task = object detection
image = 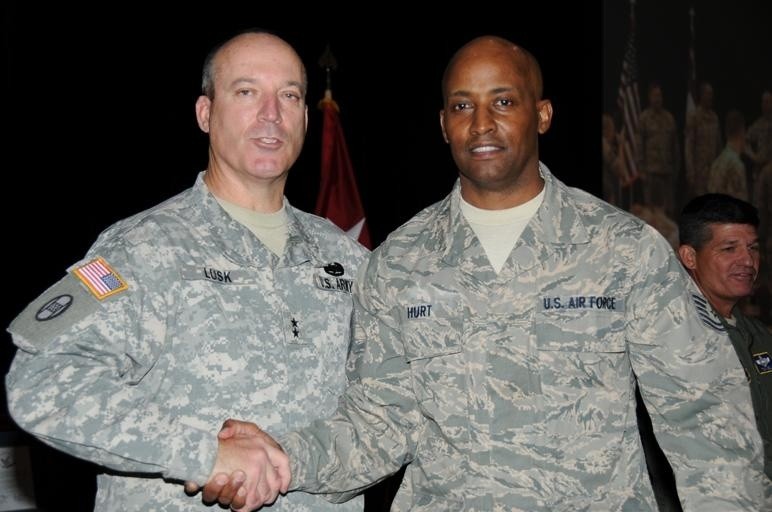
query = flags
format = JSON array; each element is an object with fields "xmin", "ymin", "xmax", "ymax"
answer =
[
  {"xmin": 309, "ymin": 87, "xmax": 373, "ymax": 250},
  {"xmin": 617, "ymin": 43, "xmax": 645, "ymax": 188}
]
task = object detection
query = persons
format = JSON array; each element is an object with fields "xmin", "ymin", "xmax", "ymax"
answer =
[
  {"xmin": 185, "ymin": 35, "xmax": 772, "ymax": 512},
  {"xmin": 638, "ymin": 191, "xmax": 772, "ymax": 511},
  {"xmin": 599, "ymin": 80, "xmax": 772, "ymax": 271},
  {"xmin": 5, "ymin": 31, "xmax": 372, "ymax": 512}
]
